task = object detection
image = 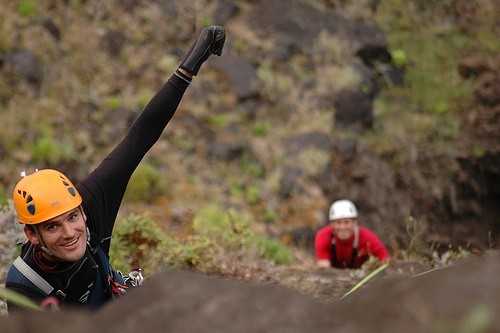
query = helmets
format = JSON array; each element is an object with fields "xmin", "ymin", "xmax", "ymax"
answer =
[
  {"xmin": 329, "ymin": 199, "xmax": 359, "ymax": 222},
  {"xmin": 13, "ymin": 168, "xmax": 83, "ymax": 224}
]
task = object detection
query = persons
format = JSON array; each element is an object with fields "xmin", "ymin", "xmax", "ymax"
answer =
[
  {"xmin": 4, "ymin": 23, "xmax": 226, "ymax": 317},
  {"xmin": 314, "ymin": 199, "xmax": 392, "ymax": 270}
]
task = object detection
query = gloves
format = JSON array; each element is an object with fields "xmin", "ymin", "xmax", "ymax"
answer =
[{"xmin": 179, "ymin": 25, "xmax": 227, "ymax": 77}]
